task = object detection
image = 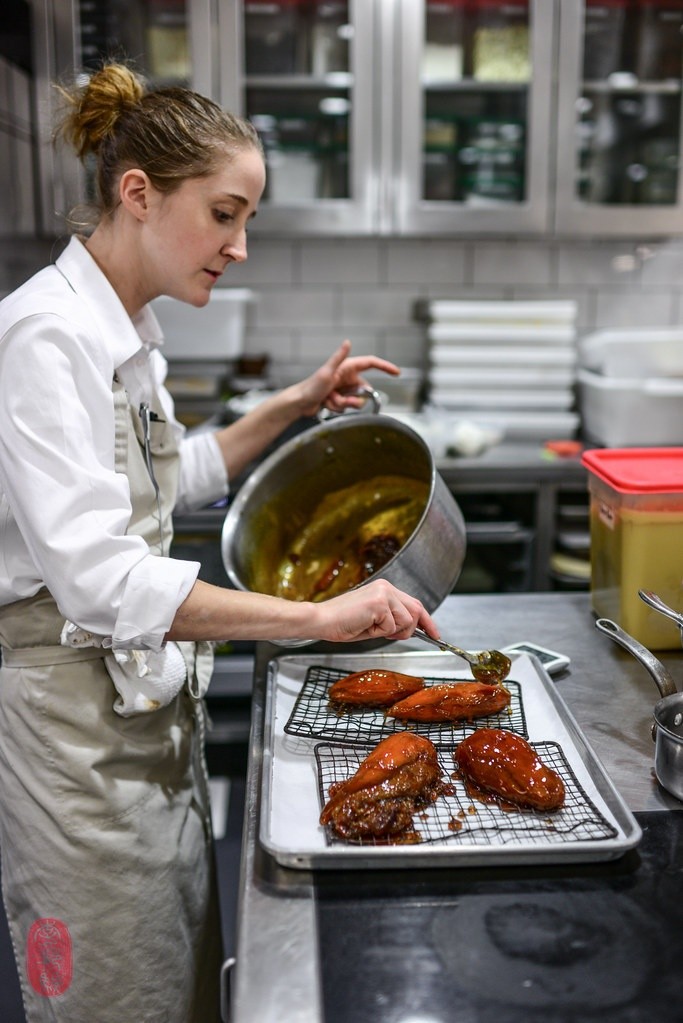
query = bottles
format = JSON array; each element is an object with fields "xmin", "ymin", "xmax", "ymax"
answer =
[
  {"xmin": 251, "ymin": 116, "xmax": 349, "ymax": 199},
  {"xmin": 470, "ymin": 125, "xmax": 521, "ymax": 196}
]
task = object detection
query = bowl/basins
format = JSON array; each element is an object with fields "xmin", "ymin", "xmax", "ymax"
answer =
[{"xmin": 227, "ymin": 384, "xmax": 387, "ymax": 463}]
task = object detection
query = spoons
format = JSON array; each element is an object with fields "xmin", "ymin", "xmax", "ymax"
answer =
[{"xmin": 413, "ymin": 628, "xmax": 511, "ymax": 685}]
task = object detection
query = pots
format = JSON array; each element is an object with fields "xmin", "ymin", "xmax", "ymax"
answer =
[
  {"xmin": 596, "ymin": 618, "xmax": 683, "ymax": 799},
  {"xmin": 220, "ymin": 386, "xmax": 467, "ymax": 649}
]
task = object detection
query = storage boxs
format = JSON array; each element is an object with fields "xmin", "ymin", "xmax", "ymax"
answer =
[
  {"xmin": 150, "ymin": 289, "xmax": 258, "ymax": 357},
  {"xmin": 581, "ymin": 328, "xmax": 683, "ymax": 648}
]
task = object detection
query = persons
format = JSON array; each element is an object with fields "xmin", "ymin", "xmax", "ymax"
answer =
[{"xmin": 1, "ymin": 64, "xmax": 448, "ymax": 1023}]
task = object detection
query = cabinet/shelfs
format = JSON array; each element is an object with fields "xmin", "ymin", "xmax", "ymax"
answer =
[
  {"xmin": 34, "ymin": 0, "xmax": 384, "ymax": 250},
  {"xmin": 376, "ymin": 0, "xmax": 683, "ymax": 241},
  {"xmin": 427, "ymin": 436, "xmax": 606, "ymax": 596}
]
